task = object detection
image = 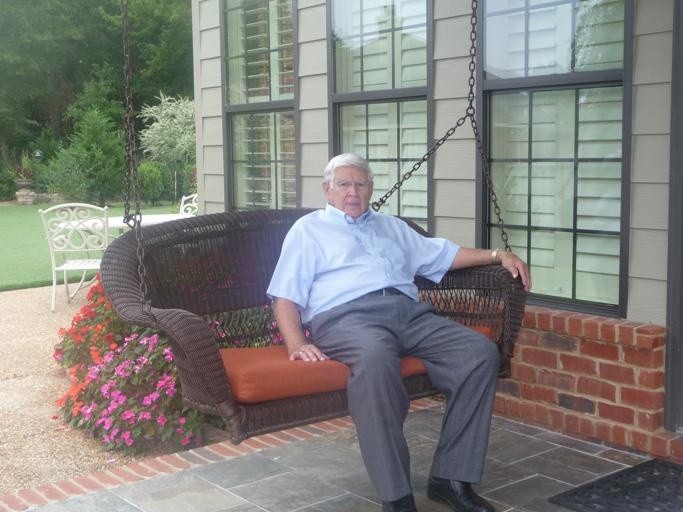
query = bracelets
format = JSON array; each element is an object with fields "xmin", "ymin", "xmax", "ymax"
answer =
[{"xmin": 492, "ymin": 246, "xmax": 503, "ymax": 265}]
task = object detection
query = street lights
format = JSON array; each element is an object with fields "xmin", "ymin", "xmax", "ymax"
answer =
[{"xmin": 31, "ymin": 150, "xmax": 44, "ymax": 202}]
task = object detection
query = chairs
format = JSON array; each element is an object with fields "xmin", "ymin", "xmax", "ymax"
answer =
[
  {"xmin": 179, "ymin": 194, "xmax": 198, "ymax": 214},
  {"xmin": 37, "ymin": 203, "xmax": 123, "ymax": 315}
]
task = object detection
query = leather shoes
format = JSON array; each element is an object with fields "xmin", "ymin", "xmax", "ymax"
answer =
[
  {"xmin": 428, "ymin": 473, "xmax": 496, "ymax": 512},
  {"xmin": 383, "ymin": 493, "xmax": 417, "ymax": 512}
]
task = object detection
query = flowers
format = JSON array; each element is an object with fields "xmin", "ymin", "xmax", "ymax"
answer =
[{"xmin": 15, "ymin": 165, "xmax": 33, "ymax": 180}]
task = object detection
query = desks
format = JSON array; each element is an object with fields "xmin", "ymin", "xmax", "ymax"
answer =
[{"xmin": 60, "ymin": 213, "xmax": 197, "ymax": 301}]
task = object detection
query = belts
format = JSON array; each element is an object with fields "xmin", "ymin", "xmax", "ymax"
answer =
[{"xmin": 366, "ymin": 287, "xmax": 403, "ymax": 297}]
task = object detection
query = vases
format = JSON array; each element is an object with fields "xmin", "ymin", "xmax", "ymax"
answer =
[{"xmin": 16, "ymin": 180, "xmax": 32, "ymax": 188}]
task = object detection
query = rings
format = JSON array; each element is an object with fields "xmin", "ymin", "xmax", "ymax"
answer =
[{"xmin": 298, "ymin": 350, "xmax": 304, "ymax": 356}]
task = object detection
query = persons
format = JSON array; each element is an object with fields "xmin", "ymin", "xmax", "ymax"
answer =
[{"xmin": 266, "ymin": 153, "xmax": 532, "ymax": 511}]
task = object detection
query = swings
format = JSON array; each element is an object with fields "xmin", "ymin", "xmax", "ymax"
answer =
[{"xmin": 98, "ymin": 0, "xmax": 529, "ymax": 445}]
